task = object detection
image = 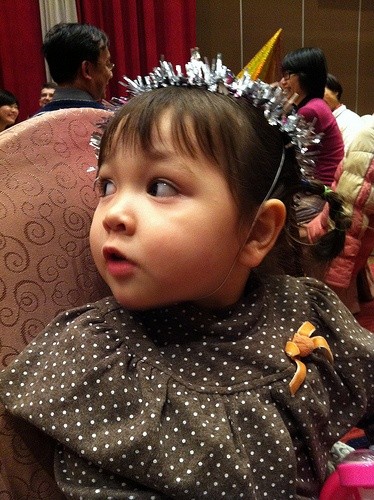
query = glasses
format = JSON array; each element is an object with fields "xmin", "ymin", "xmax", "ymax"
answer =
[
  {"xmin": 280, "ymin": 71, "xmax": 294, "ymax": 80},
  {"xmin": 91, "ymin": 60, "xmax": 115, "ymax": 71}
]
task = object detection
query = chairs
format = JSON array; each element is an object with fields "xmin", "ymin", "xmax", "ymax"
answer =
[
  {"xmin": 0, "ymin": 109, "xmax": 112, "ymax": 500},
  {"xmin": 311, "ymin": 114, "xmax": 374, "ymax": 317}
]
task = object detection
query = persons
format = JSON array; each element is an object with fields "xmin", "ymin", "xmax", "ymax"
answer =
[
  {"xmin": 1, "ymin": 22, "xmax": 374, "ymax": 226},
  {"xmin": 1, "ymin": 47, "xmax": 374, "ymax": 500}
]
